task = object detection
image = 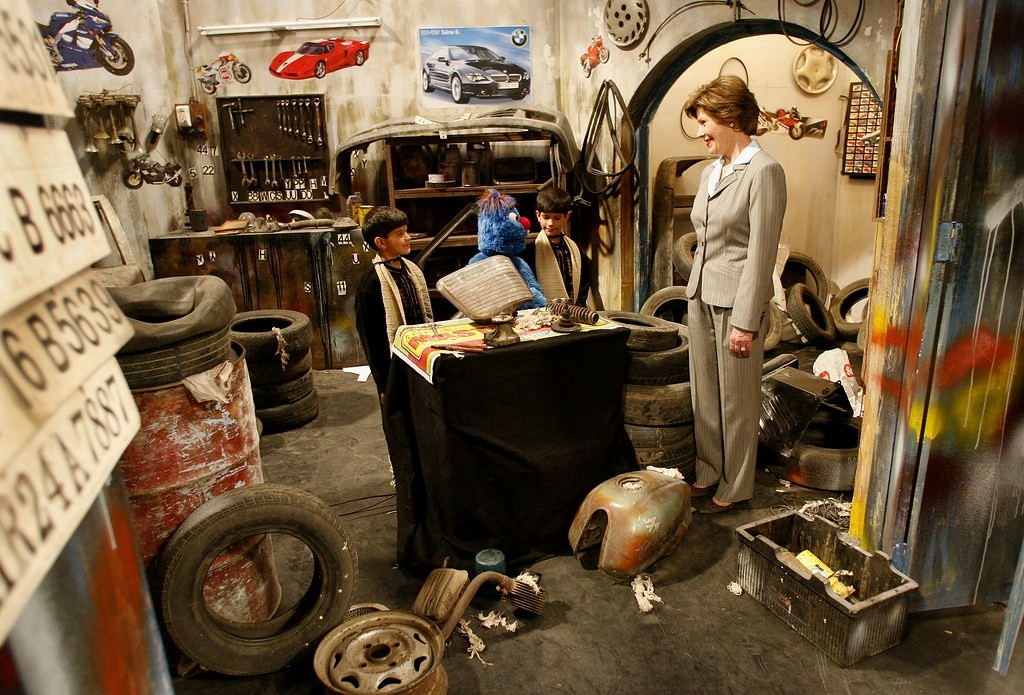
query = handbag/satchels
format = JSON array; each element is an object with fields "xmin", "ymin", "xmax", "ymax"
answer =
[{"xmin": 812, "ymin": 348, "xmax": 864, "ymax": 417}]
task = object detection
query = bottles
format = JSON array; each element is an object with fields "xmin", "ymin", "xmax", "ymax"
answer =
[
  {"xmin": 467, "ymin": 141, "xmax": 494, "ymax": 185},
  {"xmin": 437, "ymin": 144, "xmax": 460, "ymax": 182},
  {"xmin": 347, "ymin": 192, "xmax": 363, "ymax": 223},
  {"xmin": 462, "ymin": 162, "xmax": 478, "ymax": 186}
]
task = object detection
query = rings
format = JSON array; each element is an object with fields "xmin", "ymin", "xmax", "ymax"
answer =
[{"xmin": 741, "ymin": 347, "xmax": 746, "ymax": 351}]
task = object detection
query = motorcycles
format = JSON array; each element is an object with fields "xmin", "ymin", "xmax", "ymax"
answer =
[
  {"xmin": 35, "ymin": 0, "xmax": 135, "ymax": 75},
  {"xmin": 758, "ymin": 107, "xmax": 806, "ymax": 139},
  {"xmin": 123, "ymin": 155, "xmax": 182, "ymax": 189},
  {"xmin": 193, "ymin": 53, "xmax": 251, "ymax": 95},
  {"xmin": 581, "ymin": 37, "xmax": 610, "ymax": 77}
]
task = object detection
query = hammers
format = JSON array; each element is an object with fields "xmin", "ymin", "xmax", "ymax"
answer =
[{"xmin": 223, "ymin": 103, "xmax": 236, "ymax": 130}]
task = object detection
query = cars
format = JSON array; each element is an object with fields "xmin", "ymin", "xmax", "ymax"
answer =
[{"xmin": 422, "ymin": 44, "xmax": 530, "ymax": 104}]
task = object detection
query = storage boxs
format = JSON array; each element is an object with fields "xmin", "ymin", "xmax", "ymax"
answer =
[{"xmin": 735, "ymin": 510, "xmax": 921, "ymax": 667}]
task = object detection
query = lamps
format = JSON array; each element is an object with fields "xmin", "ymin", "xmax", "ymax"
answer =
[{"xmin": 196, "ymin": 16, "xmax": 381, "ymax": 36}]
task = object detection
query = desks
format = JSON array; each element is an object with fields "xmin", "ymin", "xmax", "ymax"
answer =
[{"xmin": 391, "ymin": 316, "xmax": 631, "ymax": 581}]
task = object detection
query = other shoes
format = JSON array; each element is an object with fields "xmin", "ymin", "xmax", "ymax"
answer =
[
  {"xmin": 689, "ymin": 483, "xmax": 717, "ymax": 496},
  {"xmin": 699, "ymin": 496, "xmax": 734, "ymax": 513}
]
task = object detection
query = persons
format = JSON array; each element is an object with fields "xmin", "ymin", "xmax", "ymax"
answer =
[
  {"xmin": 353, "ymin": 205, "xmax": 436, "ymax": 412},
  {"xmin": 683, "ymin": 74, "xmax": 787, "ymax": 515},
  {"xmin": 519, "ymin": 187, "xmax": 593, "ymax": 310}
]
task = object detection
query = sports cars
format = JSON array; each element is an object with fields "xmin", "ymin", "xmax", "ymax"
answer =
[{"xmin": 269, "ymin": 36, "xmax": 370, "ymax": 79}]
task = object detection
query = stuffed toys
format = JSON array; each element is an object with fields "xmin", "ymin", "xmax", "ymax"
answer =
[{"xmin": 468, "ymin": 188, "xmax": 548, "ymax": 311}]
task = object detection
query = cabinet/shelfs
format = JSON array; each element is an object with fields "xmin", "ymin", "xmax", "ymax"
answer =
[
  {"xmin": 145, "ymin": 216, "xmax": 382, "ymax": 372},
  {"xmin": 383, "ymin": 132, "xmax": 571, "ymax": 322}
]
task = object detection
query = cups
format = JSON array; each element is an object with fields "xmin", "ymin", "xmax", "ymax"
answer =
[{"xmin": 359, "ymin": 206, "xmax": 374, "ymax": 227}]
